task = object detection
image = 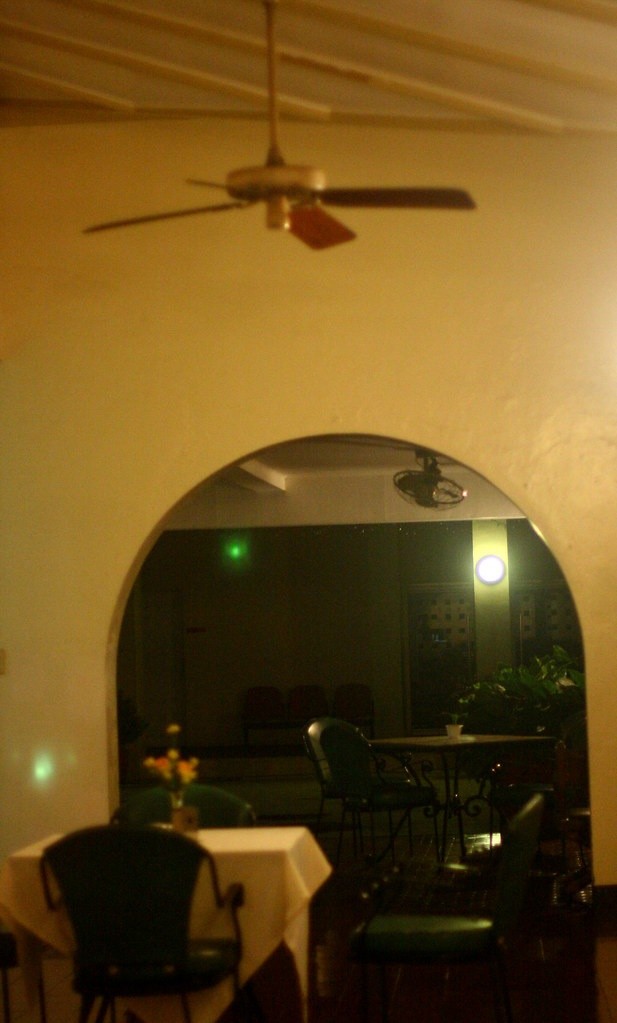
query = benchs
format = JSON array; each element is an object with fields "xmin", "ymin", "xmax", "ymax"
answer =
[{"xmin": 239, "ymin": 685, "xmax": 375, "ymax": 758}]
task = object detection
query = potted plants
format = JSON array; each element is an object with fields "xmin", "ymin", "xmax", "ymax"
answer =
[
  {"xmin": 451, "ymin": 640, "xmax": 586, "ymax": 841},
  {"xmin": 440, "ymin": 711, "xmax": 469, "ymax": 739}
]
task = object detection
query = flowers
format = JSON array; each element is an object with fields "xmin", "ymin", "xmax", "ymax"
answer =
[{"xmin": 141, "ymin": 722, "xmax": 200, "ymax": 793}]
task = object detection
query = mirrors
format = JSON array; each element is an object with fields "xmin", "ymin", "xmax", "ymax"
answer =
[{"xmin": 116, "ymin": 433, "xmax": 594, "ymax": 1023}]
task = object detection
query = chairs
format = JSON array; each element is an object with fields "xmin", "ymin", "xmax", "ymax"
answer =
[
  {"xmin": 344, "ymin": 812, "xmax": 541, "ymax": 1023},
  {"xmin": 299, "ymin": 717, "xmax": 439, "ymax": 871},
  {"xmin": 39, "ymin": 828, "xmax": 247, "ymax": 1023},
  {"xmin": 0, "ymin": 931, "xmax": 47, "ymax": 1023},
  {"xmin": 110, "ymin": 781, "xmax": 258, "ymax": 827},
  {"xmin": 484, "ymin": 722, "xmax": 582, "ymax": 862}
]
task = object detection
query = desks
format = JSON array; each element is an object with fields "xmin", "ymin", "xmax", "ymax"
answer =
[
  {"xmin": 367, "ymin": 733, "xmax": 557, "ymax": 866},
  {"xmin": 0, "ymin": 824, "xmax": 334, "ymax": 1023}
]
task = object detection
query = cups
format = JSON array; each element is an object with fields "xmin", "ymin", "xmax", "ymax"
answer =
[{"xmin": 446, "ymin": 725, "xmax": 463, "ymax": 741}]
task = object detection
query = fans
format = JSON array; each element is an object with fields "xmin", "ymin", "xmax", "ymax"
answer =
[
  {"xmin": 393, "ymin": 447, "xmax": 466, "ymax": 511},
  {"xmin": 80, "ymin": 0, "xmax": 478, "ymax": 250}
]
